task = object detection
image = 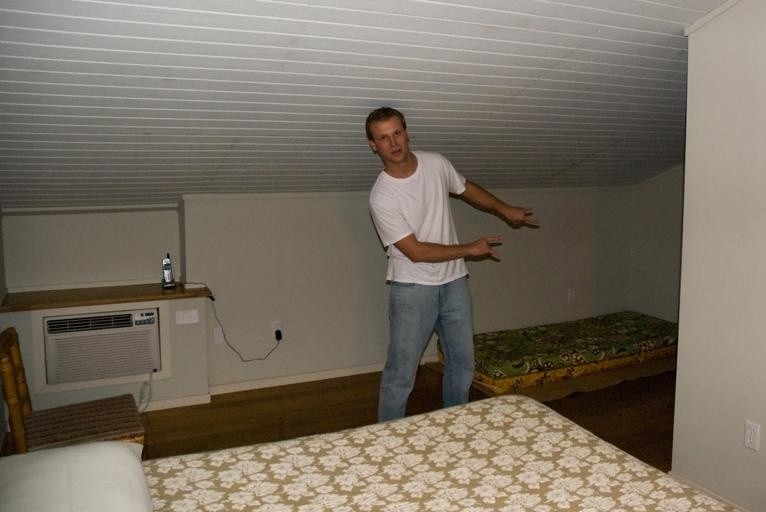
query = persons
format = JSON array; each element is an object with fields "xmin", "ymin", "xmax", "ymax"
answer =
[{"xmin": 366, "ymin": 107, "xmax": 542, "ymax": 424}]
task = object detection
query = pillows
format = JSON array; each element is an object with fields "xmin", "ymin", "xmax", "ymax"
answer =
[{"xmin": 0, "ymin": 438, "xmax": 154, "ymax": 512}]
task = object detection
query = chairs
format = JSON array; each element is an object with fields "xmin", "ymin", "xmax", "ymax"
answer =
[{"xmin": 0, "ymin": 326, "xmax": 146, "ymax": 457}]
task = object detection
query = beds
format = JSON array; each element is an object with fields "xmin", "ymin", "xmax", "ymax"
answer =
[
  {"xmin": 141, "ymin": 394, "xmax": 745, "ymax": 512},
  {"xmin": 435, "ymin": 312, "xmax": 677, "ymax": 404}
]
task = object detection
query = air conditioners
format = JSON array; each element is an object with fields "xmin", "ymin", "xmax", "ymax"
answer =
[{"xmin": 43, "ymin": 308, "xmax": 161, "ymax": 385}]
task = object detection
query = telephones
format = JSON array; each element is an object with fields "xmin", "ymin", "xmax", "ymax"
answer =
[{"xmin": 161, "ymin": 253, "xmax": 176, "ymax": 289}]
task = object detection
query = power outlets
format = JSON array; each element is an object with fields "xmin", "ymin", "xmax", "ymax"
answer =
[
  {"xmin": 745, "ymin": 420, "xmax": 761, "ymax": 450},
  {"xmin": 272, "ymin": 320, "xmax": 284, "ymax": 345}
]
